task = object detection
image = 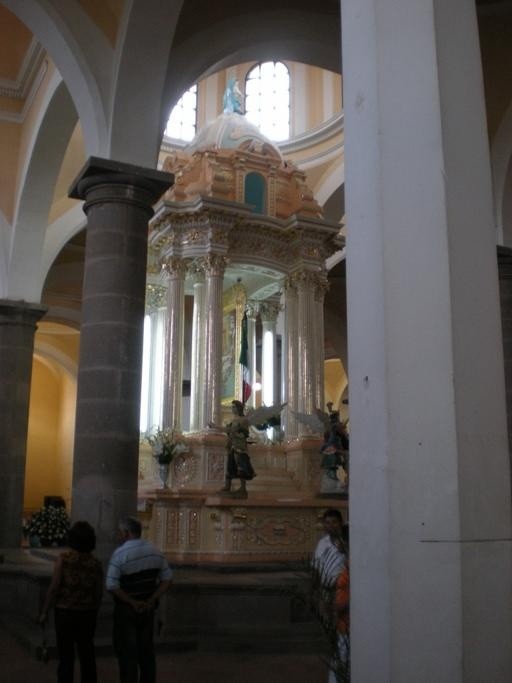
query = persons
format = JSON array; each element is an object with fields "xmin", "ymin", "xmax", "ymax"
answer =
[
  {"xmin": 309, "ymin": 505, "xmax": 341, "ymax": 583},
  {"xmin": 35, "ymin": 521, "xmax": 106, "ymax": 683},
  {"xmin": 322, "ymin": 411, "xmax": 346, "ymax": 481},
  {"xmin": 313, "ymin": 522, "xmax": 349, "ymax": 647},
  {"xmin": 208, "ymin": 399, "xmax": 255, "ymax": 498},
  {"xmin": 104, "ymin": 514, "xmax": 175, "ymax": 683}
]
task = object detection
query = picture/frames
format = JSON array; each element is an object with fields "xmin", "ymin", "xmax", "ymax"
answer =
[{"xmin": 220, "ymin": 301, "xmax": 243, "ymax": 406}]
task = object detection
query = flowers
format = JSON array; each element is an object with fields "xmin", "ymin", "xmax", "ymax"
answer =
[{"xmin": 143, "ymin": 425, "xmax": 190, "ymax": 463}]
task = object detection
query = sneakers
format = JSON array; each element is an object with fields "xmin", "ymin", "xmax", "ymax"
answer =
[{"xmin": 216, "ymin": 486, "xmax": 249, "ymax": 499}]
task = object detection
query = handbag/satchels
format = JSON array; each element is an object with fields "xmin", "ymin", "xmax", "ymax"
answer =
[
  {"xmin": 225, "ymin": 453, "xmax": 253, "ymax": 480},
  {"xmin": 37, "ymin": 562, "xmax": 72, "ymax": 609}
]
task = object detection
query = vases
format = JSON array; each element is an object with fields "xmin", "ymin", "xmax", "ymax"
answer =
[{"xmin": 153, "ymin": 454, "xmax": 173, "ymax": 489}]
